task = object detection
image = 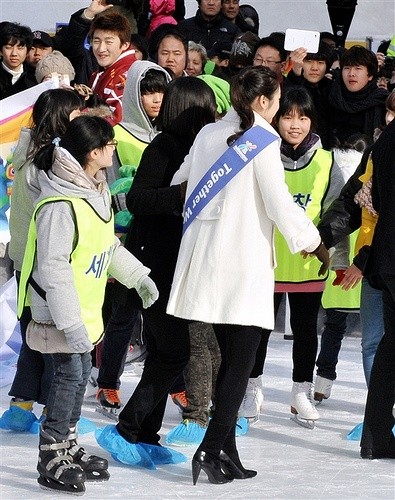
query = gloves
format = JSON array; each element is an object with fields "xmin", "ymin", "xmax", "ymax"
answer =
[
  {"xmin": 136, "ymin": 278, "xmax": 159, "ymax": 309},
  {"xmin": 332, "ymin": 270, "xmax": 347, "ymax": 286},
  {"xmin": 65, "ymin": 324, "xmax": 94, "ymax": 353}
]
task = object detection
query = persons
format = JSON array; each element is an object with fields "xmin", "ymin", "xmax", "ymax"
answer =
[
  {"xmin": 166, "ymin": 66, "xmax": 329, "ymax": 485},
  {"xmin": 95, "ymin": 76, "xmax": 220, "ymax": 471},
  {"xmin": 360, "ymin": 119, "xmax": 395, "ymax": 460},
  {"xmin": 17, "ymin": 116, "xmax": 159, "ymax": 495},
  {"xmin": 238, "ymin": 87, "xmax": 351, "ymax": 430},
  {"xmin": 313, "ymin": 137, "xmax": 374, "ymax": 406},
  {"xmin": 300, "ymin": 86, "xmax": 395, "ymax": 441},
  {"xmin": 255, "ymin": 32, "xmax": 395, "ymax": 340},
  {"xmin": 0, "ymin": 0, "xmax": 248, "ymax": 443}
]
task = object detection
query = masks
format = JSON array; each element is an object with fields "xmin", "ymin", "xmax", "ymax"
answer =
[
  {"xmin": 204, "ymin": 60, "xmax": 222, "ymax": 76},
  {"xmin": 228, "ymin": 66, "xmax": 245, "ymax": 76}
]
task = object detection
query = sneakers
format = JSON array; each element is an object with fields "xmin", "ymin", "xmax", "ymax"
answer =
[
  {"xmin": 289, "ymin": 381, "xmax": 319, "ymax": 430},
  {"xmin": 36, "ymin": 420, "xmax": 111, "ymax": 496},
  {"xmin": 171, "ymin": 391, "xmax": 188, "ymax": 419},
  {"xmin": 192, "ymin": 448, "xmax": 257, "ymax": 484},
  {"xmin": 95, "ymin": 387, "xmax": 125, "ymax": 422},
  {"xmin": 237, "ymin": 374, "xmax": 264, "ymax": 425},
  {"xmin": 314, "ymin": 375, "xmax": 334, "ymax": 405}
]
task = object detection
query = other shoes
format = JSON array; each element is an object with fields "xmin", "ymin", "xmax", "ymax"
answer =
[
  {"xmin": 10, "ymin": 398, "xmax": 35, "ymax": 431},
  {"xmin": 360, "ymin": 445, "xmax": 395, "ymax": 459}
]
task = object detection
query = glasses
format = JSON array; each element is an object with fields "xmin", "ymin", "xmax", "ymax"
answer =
[
  {"xmin": 253, "ymin": 57, "xmax": 283, "ymax": 66},
  {"xmin": 105, "ymin": 139, "xmax": 118, "ymax": 147}
]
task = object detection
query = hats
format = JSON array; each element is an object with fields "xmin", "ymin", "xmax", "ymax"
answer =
[
  {"xmin": 230, "ymin": 31, "xmax": 260, "ymax": 66},
  {"xmin": 31, "ymin": 30, "xmax": 53, "ymax": 47},
  {"xmin": 207, "ymin": 40, "xmax": 232, "ymax": 58},
  {"xmin": 35, "ymin": 50, "xmax": 75, "ymax": 83}
]
